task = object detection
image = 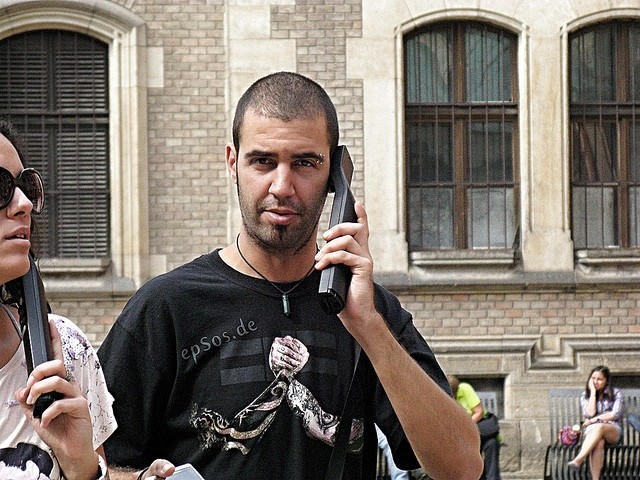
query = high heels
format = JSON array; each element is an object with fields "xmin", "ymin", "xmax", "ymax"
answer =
[{"xmin": 568, "ymin": 461, "xmax": 579, "ymax": 475}]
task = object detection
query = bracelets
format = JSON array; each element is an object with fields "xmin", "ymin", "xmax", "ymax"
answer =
[{"xmin": 136, "ymin": 466, "xmax": 149, "ymax": 480}]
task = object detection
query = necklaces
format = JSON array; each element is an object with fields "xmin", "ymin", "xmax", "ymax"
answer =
[{"xmin": 236, "ymin": 232, "xmax": 318, "ymax": 316}]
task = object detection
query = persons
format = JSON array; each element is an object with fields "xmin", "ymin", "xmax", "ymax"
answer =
[
  {"xmin": 568, "ymin": 366, "xmax": 622, "ymax": 480},
  {"xmin": 444, "ymin": 375, "xmax": 503, "ymax": 480},
  {"xmin": 1, "ymin": 117, "xmax": 108, "ymax": 480},
  {"xmin": 96, "ymin": 72, "xmax": 484, "ymax": 480}
]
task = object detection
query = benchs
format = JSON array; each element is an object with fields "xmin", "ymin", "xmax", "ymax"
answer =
[{"xmin": 543, "ymin": 389, "xmax": 639, "ymax": 476}]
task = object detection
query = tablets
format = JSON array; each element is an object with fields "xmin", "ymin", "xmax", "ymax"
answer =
[{"xmin": 138, "ymin": 462, "xmax": 205, "ymax": 480}]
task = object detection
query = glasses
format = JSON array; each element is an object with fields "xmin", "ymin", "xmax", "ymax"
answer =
[{"xmin": 0, "ymin": 167, "xmax": 44, "ymax": 215}]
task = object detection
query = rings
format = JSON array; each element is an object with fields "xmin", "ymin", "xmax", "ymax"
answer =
[{"xmin": 64, "ymin": 364, "xmax": 76, "ymax": 383}]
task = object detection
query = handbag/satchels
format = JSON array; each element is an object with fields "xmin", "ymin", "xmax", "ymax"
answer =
[
  {"xmin": 559, "ymin": 424, "xmax": 581, "ymax": 446},
  {"xmin": 477, "ymin": 412, "xmax": 500, "ymax": 439}
]
